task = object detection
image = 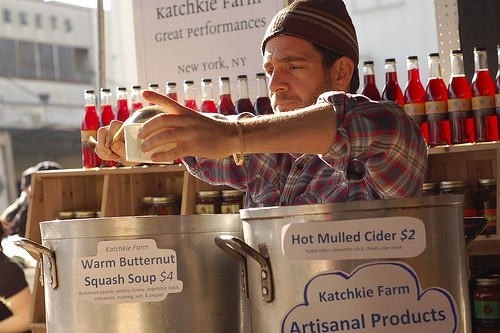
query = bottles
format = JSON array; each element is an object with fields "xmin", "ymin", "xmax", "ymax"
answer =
[
  {"xmin": 234, "ymin": 74, "xmax": 254, "ymax": 115},
  {"xmin": 141, "ymin": 194, "xmax": 180, "ymax": 215},
  {"xmin": 360, "ymin": 60, "xmax": 381, "ymax": 102},
  {"xmin": 166, "ymin": 82, "xmax": 177, "ymax": 101},
  {"xmin": 470, "ymin": 45, "xmax": 498, "ymax": 142},
  {"xmin": 253, "ymin": 72, "xmax": 274, "ymax": 115},
  {"xmin": 183, "ymin": 80, "xmax": 199, "ymax": 112},
  {"xmin": 494, "ymin": 45, "xmax": 500, "ymax": 140},
  {"xmin": 381, "ymin": 58, "xmax": 404, "ymax": 105},
  {"xmin": 199, "ymin": 78, "xmax": 218, "ymax": 113},
  {"xmin": 425, "ymin": 53, "xmax": 451, "ymax": 147},
  {"xmin": 420, "ymin": 177, "xmax": 500, "ymax": 333},
  {"xmin": 81, "ymin": 84, "xmax": 159, "ymax": 168},
  {"xmin": 447, "ymin": 49, "xmax": 475, "ymax": 145},
  {"xmin": 403, "ymin": 55, "xmax": 430, "ymax": 147},
  {"xmin": 216, "ymin": 77, "xmax": 234, "ymax": 115},
  {"xmin": 194, "ymin": 189, "xmax": 244, "ymax": 213}
]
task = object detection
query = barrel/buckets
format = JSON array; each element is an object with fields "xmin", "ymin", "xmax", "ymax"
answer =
[
  {"xmin": 214, "ymin": 192, "xmax": 488, "ymax": 333},
  {"xmin": 12, "ymin": 213, "xmax": 240, "ymax": 333}
]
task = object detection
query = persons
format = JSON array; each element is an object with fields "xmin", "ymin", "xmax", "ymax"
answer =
[
  {"xmin": 94, "ymin": 0, "xmax": 427, "ymax": 208},
  {"xmin": 0, "ymin": 161, "xmax": 64, "ymax": 333}
]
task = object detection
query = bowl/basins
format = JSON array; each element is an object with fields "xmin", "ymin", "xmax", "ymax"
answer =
[{"xmin": 123, "ymin": 122, "xmax": 174, "ymax": 166}]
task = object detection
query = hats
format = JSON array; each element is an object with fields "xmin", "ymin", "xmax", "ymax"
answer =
[{"xmin": 261, "ymin": 0, "xmax": 359, "ymax": 69}]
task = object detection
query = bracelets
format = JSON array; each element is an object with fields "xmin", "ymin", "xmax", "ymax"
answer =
[{"xmin": 232, "ymin": 121, "xmax": 244, "ymax": 165}]
